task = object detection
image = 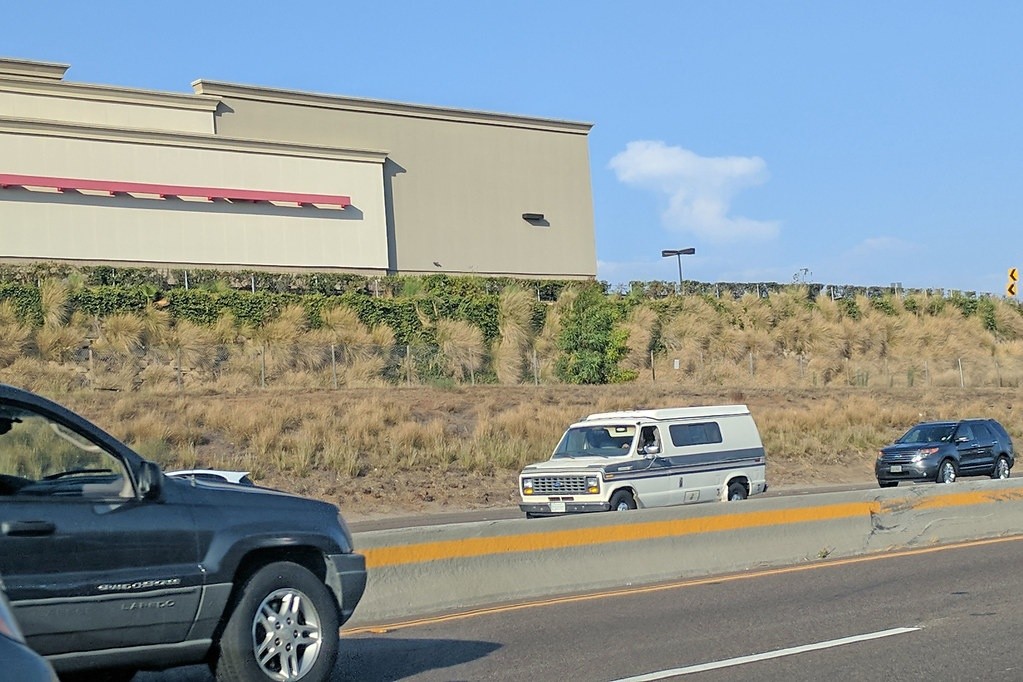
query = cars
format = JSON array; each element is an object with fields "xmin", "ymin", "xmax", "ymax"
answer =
[{"xmin": 0, "ymin": 578, "xmax": 59, "ymax": 679}]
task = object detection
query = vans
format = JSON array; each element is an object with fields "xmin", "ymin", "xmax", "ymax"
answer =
[{"xmin": 517, "ymin": 403, "xmax": 769, "ymax": 520}]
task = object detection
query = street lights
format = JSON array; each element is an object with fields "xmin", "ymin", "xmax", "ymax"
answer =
[{"xmin": 662, "ymin": 247, "xmax": 694, "ymax": 292}]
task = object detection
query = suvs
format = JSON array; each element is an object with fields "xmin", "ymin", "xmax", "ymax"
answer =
[
  {"xmin": 875, "ymin": 418, "xmax": 1016, "ymax": 489},
  {"xmin": 1, "ymin": 384, "xmax": 367, "ymax": 682}
]
